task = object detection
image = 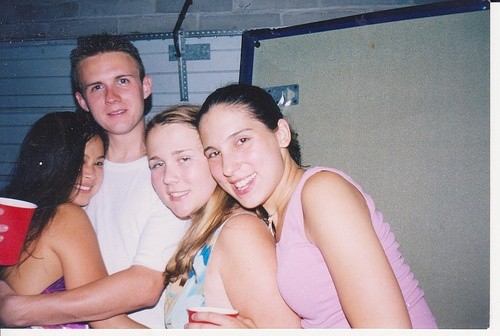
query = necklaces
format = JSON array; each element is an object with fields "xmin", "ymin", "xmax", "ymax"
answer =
[{"xmin": 266, "ymin": 166, "xmax": 297, "ymax": 222}]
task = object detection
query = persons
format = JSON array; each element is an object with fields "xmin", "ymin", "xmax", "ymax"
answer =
[
  {"xmin": 0, "ymin": 33, "xmax": 192, "ymax": 328},
  {"xmin": 142, "ymin": 106, "xmax": 302, "ymax": 329},
  {"xmin": 0, "ymin": 112, "xmax": 152, "ymax": 329},
  {"xmin": 184, "ymin": 85, "xmax": 439, "ymax": 330}
]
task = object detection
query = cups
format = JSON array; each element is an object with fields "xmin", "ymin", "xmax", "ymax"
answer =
[
  {"xmin": 0, "ymin": 198, "xmax": 39, "ymax": 266},
  {"xmin": 186, "ymin": 308, "xmax": 239, "ymax": 325}
]
